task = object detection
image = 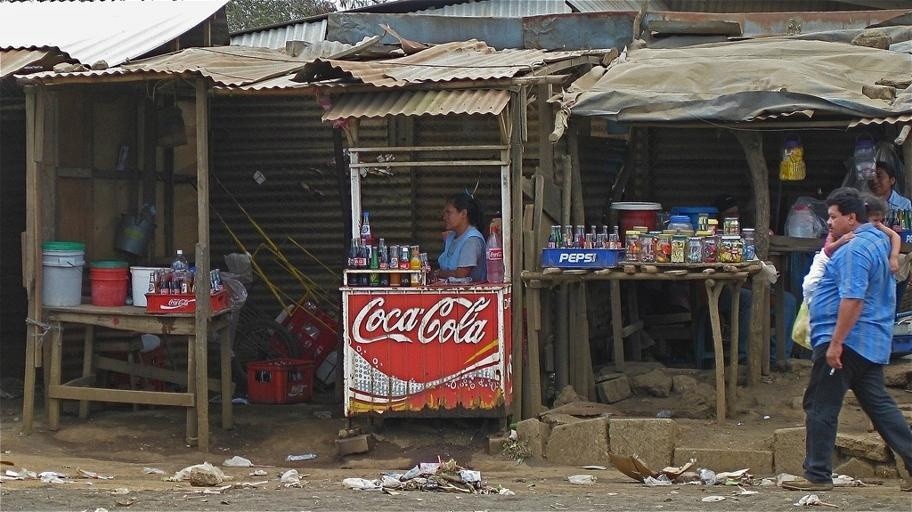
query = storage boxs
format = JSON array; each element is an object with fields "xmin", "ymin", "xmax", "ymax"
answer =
[
  {"xmin": 540, "ymin": 248, "xmax": 624, "ymax": 269},
  {"xmin": 271, "ymin": 302, "xmax": 338, "ymax": 370},
  {"xmin": 144, "ymin": 289, "xmax": 229, "ymax": 314},
  {"xmin": 248, "ymin": 358, "xmax": 315, "ymax": 403}
]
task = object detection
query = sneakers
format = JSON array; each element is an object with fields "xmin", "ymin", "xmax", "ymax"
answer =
[
  {"xmin": 900, "ymin": 480, "xmax": 911, "ymax": 492},
  {"xmin": 782, "ymin": 479, "xmax": 833, "ymax": 491}
]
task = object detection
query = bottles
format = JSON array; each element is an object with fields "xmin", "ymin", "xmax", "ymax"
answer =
[
  {"xmin": 624, "ymin": 213, "xmax": 755, "ymax": 263},
  {"xmin": 883, "ymin": 208, "xmax": 912, "ymax": 230},
  {"xmin": 547, "ymin": 225, "xmax": 622, "ymax": 249},
  {"xmin": 256, "ymin": 359, "xmax": 303, "ymax": 383},
  {"xmin": 149, "ymin": 249, "xmax": 224, "ymax": 296},
  {"xmin": 853, "ymin": 139, "xmax": 877, "ymax": 181},
  {"xmin": 303, "ymin": 301, "xmax": 321, "ymax": 338},
  {"xmin": 486, "ymin": 227, "xmax": 503, "ymax": 284},
  {"xmin": 348, "ymin": 212, "xmax": 431, "ymax": 286},
  {"xmin": 788, "ymin": 205, "xmax": 816, "ymax": 238}
]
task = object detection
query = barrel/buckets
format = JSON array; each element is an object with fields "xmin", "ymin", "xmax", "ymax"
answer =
[
  {"xmin": 90, "ymin": 268, "xmax": 129, "ymax": 307},
  {"xmin": 42, "ymin": 261, "xmax": 84, "ymax": 307},
  {"xmin": 42, "ymin": 241, "xmax": 85, "ymax": 251},
  {"xmin": 42, "ymin": 251, "xmax": 84, "ymax": 261},
  {"xmin": 670, "ymin": 205, "xmax": 719, "ymax": 230},
  {"xmin": 131, "ymin": 267, "xmax": 170, "ymax": 307},
  {"xmin": 609, "ymin": 202, "xmax": 663, "ymax": 249},
  {"xmin": 90, "ymin": 260, "xmax": 130, "ymax": 268}
]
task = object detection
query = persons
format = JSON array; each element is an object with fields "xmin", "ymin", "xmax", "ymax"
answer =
[
  {"xmin": 701, "ymin": 194, "xmax": 798, "ymax": 371},
  {"xmin": 868, "ymin": 161, "xmax": 911, "ymax": 210},
  {"xmin": 802, "ymin": 193, "xmax": 902, "ymax": 312},
  {"xmin": 431, "ymin": 194, "xmax": 488, "ymax": 282},
  {"xmin": 782, "ymin": 186, "xmax": 912, "ymax": 490}
]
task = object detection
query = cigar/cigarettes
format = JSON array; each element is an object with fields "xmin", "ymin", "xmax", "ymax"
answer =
[{"xmin": 829, "ymin": 368, "xmax": 836, "ymax": 376}]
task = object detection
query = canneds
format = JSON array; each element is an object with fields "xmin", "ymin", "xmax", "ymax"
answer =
[
  {"xmin": 671, "ymin": 235, "xmax": 686, "ymax": 263},
  {"xmin": 719, "ymin": 236, "xmax": 744, "ymax": 263},
  {"xmin": 684, "ymin": 237, "xmax": 703, "ymax": 264},
  {"xmin": 702, "ymin": 236, "xmax": 719, "ymax": 263},
  {"xmin": 741, "ymin": 228, "xmax": 756, "ymax": 238},
  {"xmin": 724, "ymin": 217, "xmax": 740, "ymax": 236}
]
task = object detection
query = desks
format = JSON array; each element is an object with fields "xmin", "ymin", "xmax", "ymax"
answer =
[
  {"xmin": 520, "ymin": 266, "xmax": 760, "ymax": 424},
  {"xmin": 759, "ymin": 235, "xmax": 911, "ymax": 373},
  {"xmin": 40, "ymin": 303, "xmax": 234, "ymax": 445}
]
